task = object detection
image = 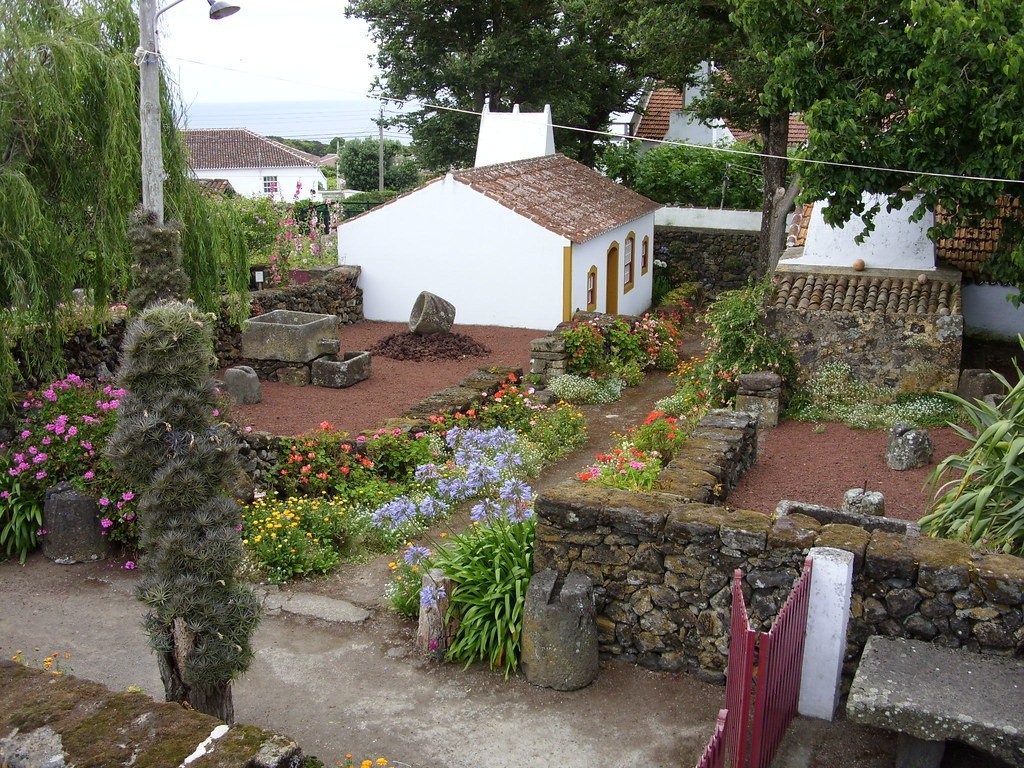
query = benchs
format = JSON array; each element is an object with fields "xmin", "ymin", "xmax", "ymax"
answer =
[{"xmin": 845, "ymin": 635, "xmax": 1023, "ymax": 768}]
